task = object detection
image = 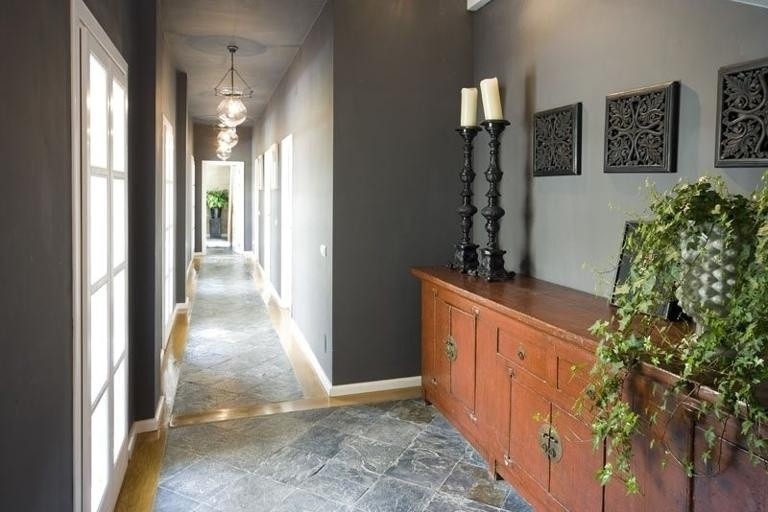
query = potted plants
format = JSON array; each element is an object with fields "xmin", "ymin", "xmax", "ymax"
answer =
[
  {"xmin": 530, "ymin": 173, "xmax": 768, "ymax": 498},
  {"xmin": 206, "ymin": 188, "xmax": 229, "ymax": 219}
]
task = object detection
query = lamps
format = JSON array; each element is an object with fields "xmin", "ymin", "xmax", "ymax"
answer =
[{"xmin": 212, "ymin": 44, "xmax": 253, "ymax": 161}]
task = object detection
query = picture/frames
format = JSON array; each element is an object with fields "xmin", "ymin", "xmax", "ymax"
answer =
[{"xmin": 609, "ymin": 220, "xmax": 673, "ymax": 322}]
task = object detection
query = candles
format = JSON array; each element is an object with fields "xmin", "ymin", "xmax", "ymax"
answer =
[
  {"xmin": 480, "ymin": 76, "xmax": 503, "ymax": 120},
  {"xmin": 460, "ymin": 87, "xmax": 478, "ymax": 128}
]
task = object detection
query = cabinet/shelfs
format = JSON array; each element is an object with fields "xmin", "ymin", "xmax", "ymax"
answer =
[{"xmin": 408, "ymin": 265, "xmax": 768, "ymax": 512}]
